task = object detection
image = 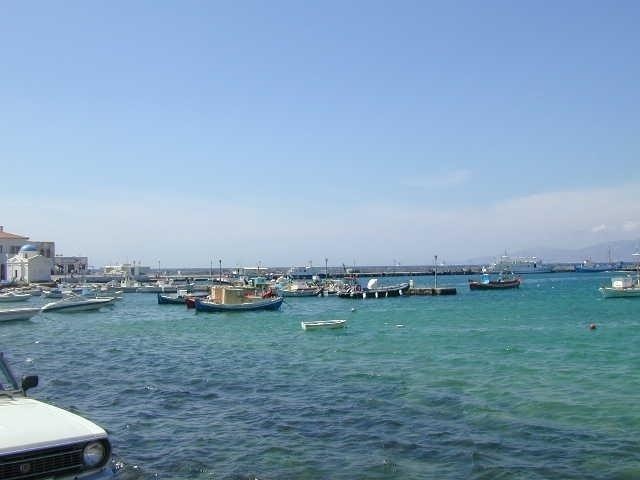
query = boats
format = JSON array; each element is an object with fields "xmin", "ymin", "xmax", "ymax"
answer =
[
  {"xmin": 600, "ymin": 277, "xmax": 639, "ymax": 298},
  {"xmin": 269, "ymin": 279, "xmax": 409, "ymax": 298},
  {"xmin": 157, "ymin": 293, "xmax": 285, "ymax": 313},
  {"xmin": 0, "ymin": 285, "xmax": 123, "ymax": 322},
  {"xmin": 301, "ymin": 320, "xmax": 347, "ymax": 330},
  {"xmin": 468, "ymin": 276, "xmax": 521, "ymax": 290}
]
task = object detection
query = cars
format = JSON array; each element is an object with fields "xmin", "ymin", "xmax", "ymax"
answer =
[{"xmin": 0, "ymin": 350, "xmax": 113, "ymax": 480}]
